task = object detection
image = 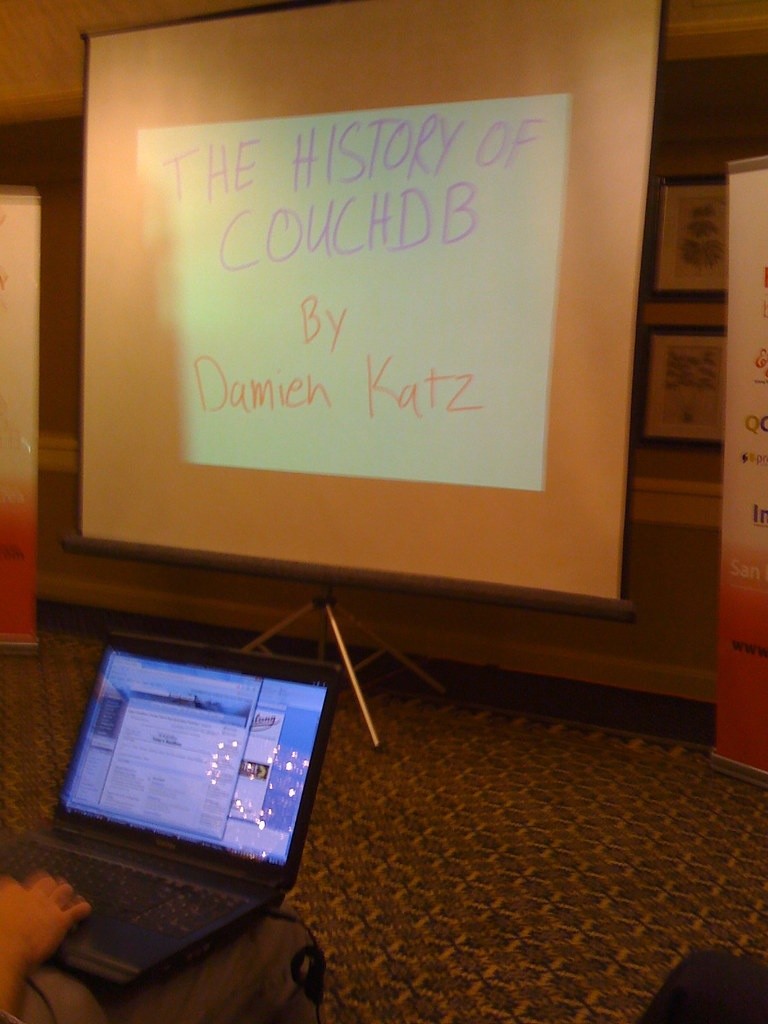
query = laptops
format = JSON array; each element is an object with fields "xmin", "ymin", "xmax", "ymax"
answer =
[{"xmin": 0, "ymin": 636, "xmax": 343, "ymax": 988}]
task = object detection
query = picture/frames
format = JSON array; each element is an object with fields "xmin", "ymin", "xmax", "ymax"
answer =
[
  {"xmin": 648, "ymin": 173, "xmax": 729, "ymax": 303},
  {"xmin": 635, "ymin": 323, "xmax": 727, "ymax": 454}
]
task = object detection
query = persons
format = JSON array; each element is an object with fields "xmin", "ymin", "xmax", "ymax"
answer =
[{"xmin": 0, "ymin": 868, "xmax": 322, "ymax": 1024}]
